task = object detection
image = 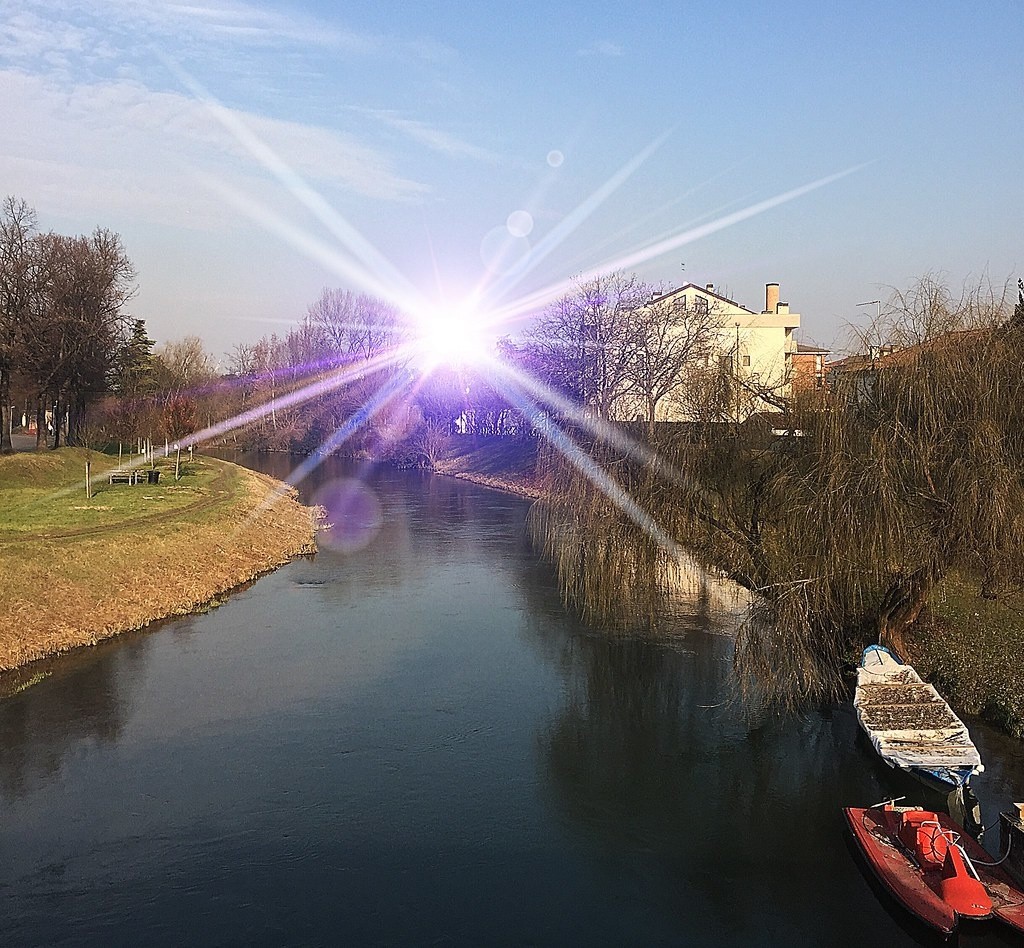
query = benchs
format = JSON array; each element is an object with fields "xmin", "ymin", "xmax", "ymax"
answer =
[{"xmin": 108, "ymin": 469, "xmax": 148, "ymax": 485}]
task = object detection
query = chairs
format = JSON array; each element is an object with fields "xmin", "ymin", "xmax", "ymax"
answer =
[
  {"xmin": 916, "ymin": 826, "xmax": 954, "ymax": 868},
  {"xmin": 902, "ymin": 810, "xmax": 938, "ymax": 849}
]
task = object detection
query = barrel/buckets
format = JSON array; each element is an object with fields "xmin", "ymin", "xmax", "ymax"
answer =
[{"xmin": 147, "ymin": 471, "xmax": 160, "ymax": 484}]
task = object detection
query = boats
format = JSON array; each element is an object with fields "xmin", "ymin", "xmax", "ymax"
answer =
[
  {"xmin": 843, "ymin": 796, "xmax": 1024, "ymax": 948},
  {"xmin": 854, "ymin": 644, "xmax": 984, "ymax": 796}
]
